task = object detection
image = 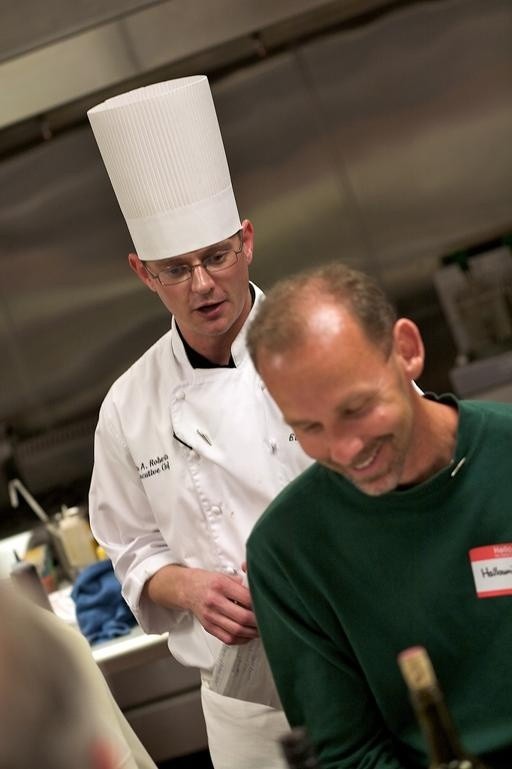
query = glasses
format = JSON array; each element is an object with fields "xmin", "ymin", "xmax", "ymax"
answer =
[{"xmin": 144, "ymin": 241, "xmax": 242, "ymax": 287}]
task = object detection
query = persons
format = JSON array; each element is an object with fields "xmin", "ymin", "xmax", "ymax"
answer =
[
  {"xmin": 87, "ymin": 220, "xmax": 427, "ymax": 769},
  {"xmin": 245, "ymin": 260, "xmax": 512, "ymax": 769}
]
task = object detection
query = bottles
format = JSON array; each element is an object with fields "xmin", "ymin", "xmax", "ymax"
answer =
[
  {"xmin": 398, "ymin": 645, "xmax": 480, "ymax": 769},
  {"xmin": 10, "ymin": 550, "xmax": 55, "ymax": 614},
  {"xmin": 44, "ymin": 505, "xmax": 100, "ymax": 582}
]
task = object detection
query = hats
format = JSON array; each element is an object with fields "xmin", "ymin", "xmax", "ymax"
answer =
[{"xmin": 86, "ymin": 74, "xmax": 243, "ymax": 261}]
